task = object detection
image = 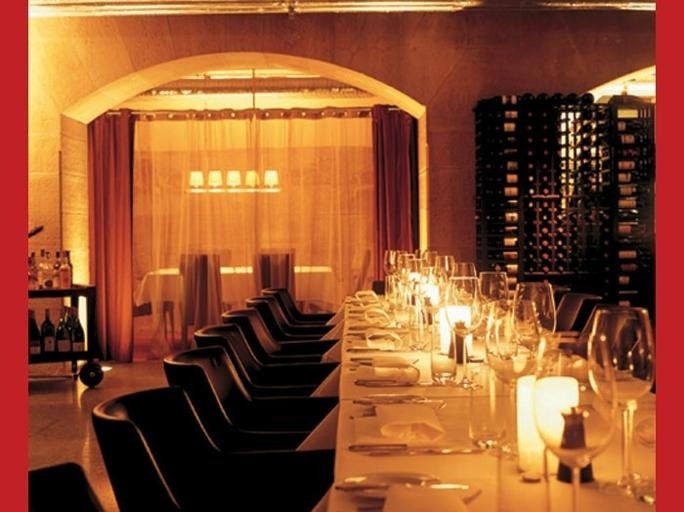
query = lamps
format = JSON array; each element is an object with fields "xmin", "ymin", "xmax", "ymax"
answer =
[{"xmin": 176, "ymin": 66, "xmax": 281, "ymax": 197}]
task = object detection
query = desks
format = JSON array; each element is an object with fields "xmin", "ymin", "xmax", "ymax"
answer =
[
  {"xmin": 156, "ymin": 267, "xmax": 334, "ymax": 343},
  {"xmin": 331, "ymin": 295, "xmax": 655, "ymax": 512}
]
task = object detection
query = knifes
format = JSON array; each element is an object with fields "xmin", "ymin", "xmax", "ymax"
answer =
[
  {"xmin": 333, "ymin": 485, "xmax": 472, "ymax": 491},
  {"xmin": 367, "ymin": 447, "xmax": 486, "ymax": 458}
]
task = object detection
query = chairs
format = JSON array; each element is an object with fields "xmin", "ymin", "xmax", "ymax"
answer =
[
  {"xmin": 28, "ymin": 287, "xmax": 340, "ymax": 512},
  {"xmin": 181, "ymin": 253, "xmax": 232, "ymax": 343},
  {"xmin": 256, "ymin": 247, "xmax": 295, "ymax": 300}
]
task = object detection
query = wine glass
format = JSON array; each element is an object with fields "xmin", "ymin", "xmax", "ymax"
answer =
[
  {"xmin": 533, "ymin": 332, "xmax": 620, "ymax": 511},
  {"xmin": 583, "ymin": 306, "xmax": 656, "ymax": 501},
  {"xmin": 382, "ymin": 247, "xmax": 565, "ymax": 437}
]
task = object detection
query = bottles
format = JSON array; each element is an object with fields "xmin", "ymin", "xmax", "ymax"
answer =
[
  {"xmin": 28, "ymin": 249, "xmax": 73, "ymax": 290},
  {"xmin": 29, "ymin": 303, "xmax": 85, "ymax": 355},
  {"xmin": 476, "ymin": 93, "xmax": 652, "ymax": 307}
]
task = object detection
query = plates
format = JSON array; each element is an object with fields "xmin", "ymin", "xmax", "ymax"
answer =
[
  {"xmin": 338, "ymin": 470, "xmax": 443, "ymax": 501},
  {"xmin": 345, "ymin": 308, "xmax": 403, "ymax": 388}
]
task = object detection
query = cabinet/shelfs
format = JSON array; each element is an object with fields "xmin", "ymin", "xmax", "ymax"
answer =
[{"xmin": 472, "ymin": 91, "xmax": 654, "ymax": 342}]
